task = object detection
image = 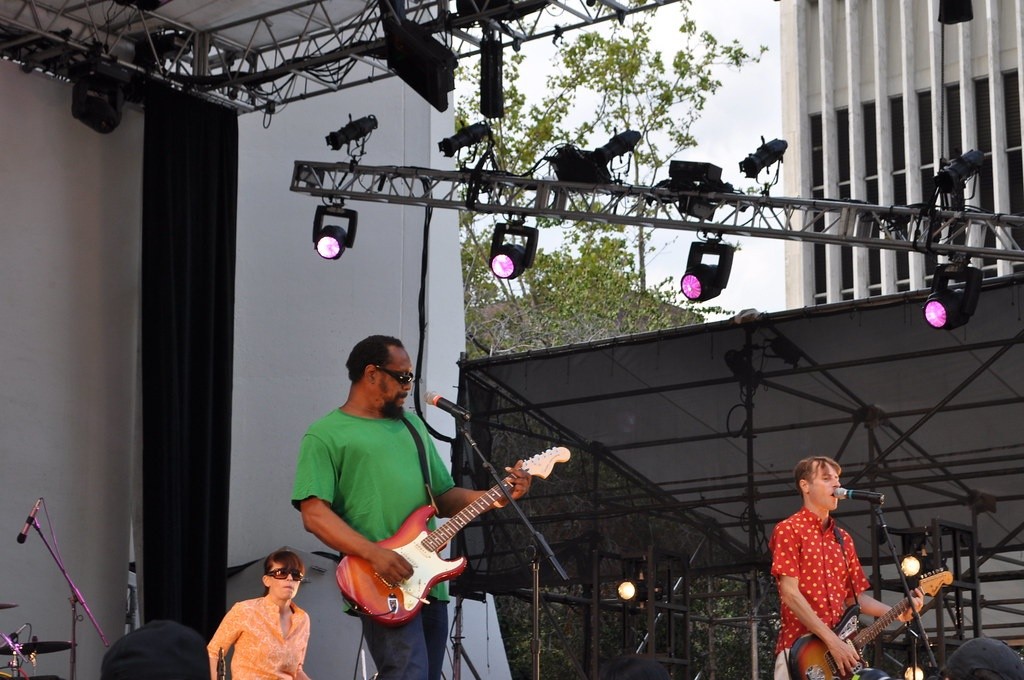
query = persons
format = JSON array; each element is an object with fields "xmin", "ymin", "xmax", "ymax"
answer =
[
  {"xmin": 289, "ymin": 335, "xmax": 531, "ymax": 680},
  {"xmin": 598, "ymin": 636, "xmax": 1024, "ymax": 680},
  {"xmin": 768, "ymin": 456, "xmax": 923, "ymax": 680},
  {"xmin": 102, "ymin": 619, "xmax": 212, "ymax": 680},
  {"xmin": 207, "ymin": 550, "xmax": 311, "ymax": 680}
]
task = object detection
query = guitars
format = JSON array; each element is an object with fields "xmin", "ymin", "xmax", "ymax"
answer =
[
  {"xmin": 789, "ymin": 567, "xmax": 953, "ymax": 680},
  {"xmin": 335, "ymin": 447, "xmax": 571, "ymax": 628}
]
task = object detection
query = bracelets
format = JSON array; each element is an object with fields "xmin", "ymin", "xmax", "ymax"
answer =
[
  {"xmin": 493, "ymin": 501, "xmax": 503, "ymax": 508},
  {"xmin": 898, "ymin": 612, "xmax": 905, "ymax": 623}
]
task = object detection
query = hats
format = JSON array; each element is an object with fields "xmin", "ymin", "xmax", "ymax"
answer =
[
  {"xmin": 101, "ymin": 621, "xmax": 210, "ymax": 680},
  {"xmin": 946, "ymin": 638, "xmax": 1024, "ymax": 680}
]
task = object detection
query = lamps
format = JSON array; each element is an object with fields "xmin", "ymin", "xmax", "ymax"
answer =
[
  {"xmin": 616, "ymin": 579, "xmax": 663, "ymax": 604},
  {"xmin": 592, "ymin": 130, "xmax": 641, "ymax": 163},
  {"xmin": 740, "ymin": 136, "xmax": 790, "ymax": 191},
  {"xmin": 900, "ymin": 554, "xmax": 947, "ymax": 577},
  {"xmin": 681, "ymin": 241, "xmax": 738, "ymax": 301},
  {"xmin": 488, "ymin": 225, "xmax": 540, "ymax": 281},
  {"xmin": 934, "ymin": 147, "xmax": 988, "ymax": 193},
  {"xmin": 326, "ymin": 114, "xmax": 379, "ymax": 152},
  {"xmin": 906, "ymin": 666, "xmax": 943, "ymax": 680},
  {"xmin": 439, "ymin": 125, "xmax": 489, "ymax": 158},
  {"xmin": 72, "ymin": 74, "xmax": 126, "ymax": 133},
  {"xmin": 924, "ymin": 261, "xmax": 986, "ymax": 331},
  {"xmin": 384, "ymin": 18, "xmax": 459, "ymax": 110},
  {"xmin": 309, "ymin": 205, "xmax": 361, "ymax": 259}
]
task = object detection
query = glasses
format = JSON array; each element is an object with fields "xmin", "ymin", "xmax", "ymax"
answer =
[
  {"xmin": 375, "ymin": 365, "xmax": 413, "ymax": 384},
  {"xmin": 267, "ymin": 568, "xmax": 303, "ymax": 580}
]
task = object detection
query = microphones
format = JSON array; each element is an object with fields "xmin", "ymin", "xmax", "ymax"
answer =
[
  {"xmin": 17, "ymin": 499, "xmax": 41, "ymax": 544},
  {"xmin": 424, "ymin": 392, "xmax": 472, "ymax": 422},
  {"xmin": 217, "ymin": 647, "xmax": 226, "ymax": 680},
  {"xmin": 834, "ymin": 488, "xmax": 884, "ymax": 503},
  {"xmin": 0, "ymin": 622, "xmax": 26, "ymax": 648}
]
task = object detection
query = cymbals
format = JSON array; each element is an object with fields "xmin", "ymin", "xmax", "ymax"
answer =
[
  {"xmin": 0, "ymin": 603, "xmax": 20, "ymax": 609},
  {"xmin": 0, "ymin": 641, "xmax": 78, "ymax": 656}
]
